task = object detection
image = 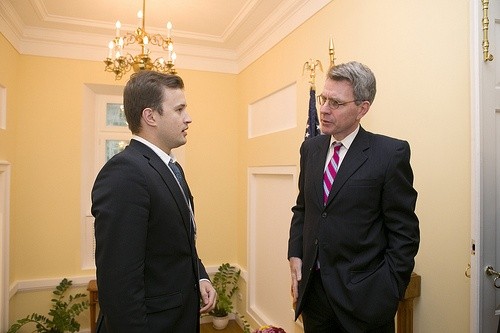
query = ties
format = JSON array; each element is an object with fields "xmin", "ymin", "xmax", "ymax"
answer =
[
  {"xmin": 323, "ymin": 142, "xmax": 343, "ymax": 207},
  {"xmin": 168, "ymin": 162, "xmax": 189, "ymax": 205}
]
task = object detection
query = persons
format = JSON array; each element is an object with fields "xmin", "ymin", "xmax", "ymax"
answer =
[
  {"xmin": 91, "ymin": 70, "xmax": 218, "ymax": 332},
  {"xmin": 288, "ymin": 61, "xmax": 420, "ymax": 333}
]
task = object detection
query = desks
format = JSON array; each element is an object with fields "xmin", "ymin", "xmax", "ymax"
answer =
[{"xmin": 87, "ymin": 280, "xmax": 99, "ymax": 333}]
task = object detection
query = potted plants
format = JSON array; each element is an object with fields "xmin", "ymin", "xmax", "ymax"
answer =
[{"xmin": 200, "ymin": 263, "xmax": 251, "ymax": 333}]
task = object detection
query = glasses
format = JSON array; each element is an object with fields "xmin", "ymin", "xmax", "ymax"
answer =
[{"xmin": 317, "ymin": 94, "xmax": 369, "ymax": 110}]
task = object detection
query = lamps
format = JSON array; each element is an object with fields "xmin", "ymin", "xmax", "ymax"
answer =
[{"xmin": 104, "ymin": 0, "xmax": 177, "ymax": 80}]
